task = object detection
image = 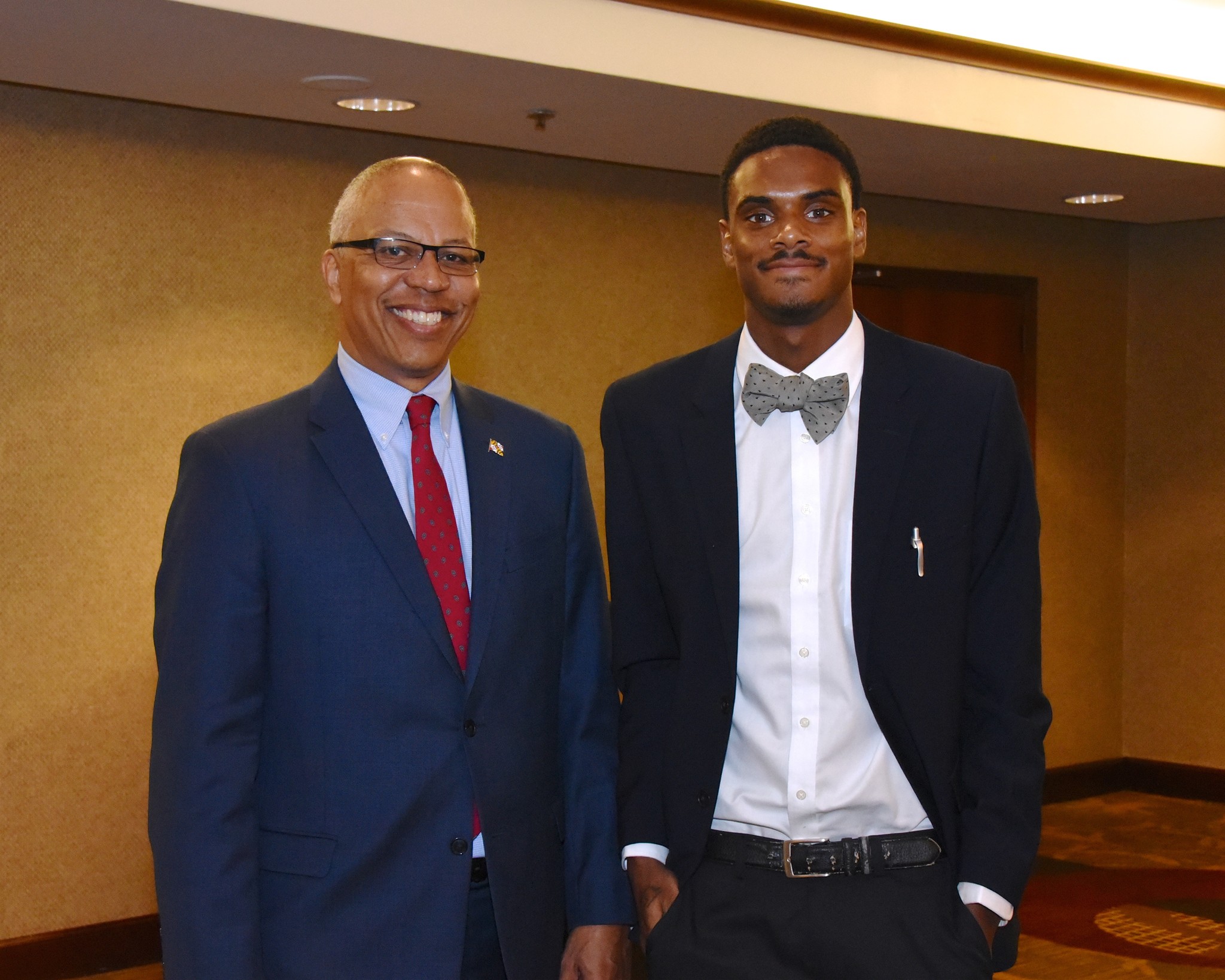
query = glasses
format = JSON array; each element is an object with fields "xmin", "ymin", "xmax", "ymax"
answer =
[{"xmin": 330, "ymin": 237, "xmax": 486, "ymax": 276}]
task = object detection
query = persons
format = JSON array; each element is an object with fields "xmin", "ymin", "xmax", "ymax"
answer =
[
  {"xmin": 599, "ymin": 115, "xmax": 1054, "ymax": 979},
  {"xmin": 145, "ymin": 156, "xmax": 638, "ymax": 979}
]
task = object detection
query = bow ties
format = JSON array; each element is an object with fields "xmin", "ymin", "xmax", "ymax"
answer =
[{"xmin": 741, "ymin": 363, "xmax": 850, "ymax": 445}]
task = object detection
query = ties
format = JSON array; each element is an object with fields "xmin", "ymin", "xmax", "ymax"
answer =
[{"xmin": 405, "ymin": 395, "xmax": 483, "ymax": 841}]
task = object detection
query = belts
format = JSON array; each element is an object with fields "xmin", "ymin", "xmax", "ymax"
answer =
[{"xmin": 703, "ymin": 829, "xmax": 944, "ymax": 877}]
